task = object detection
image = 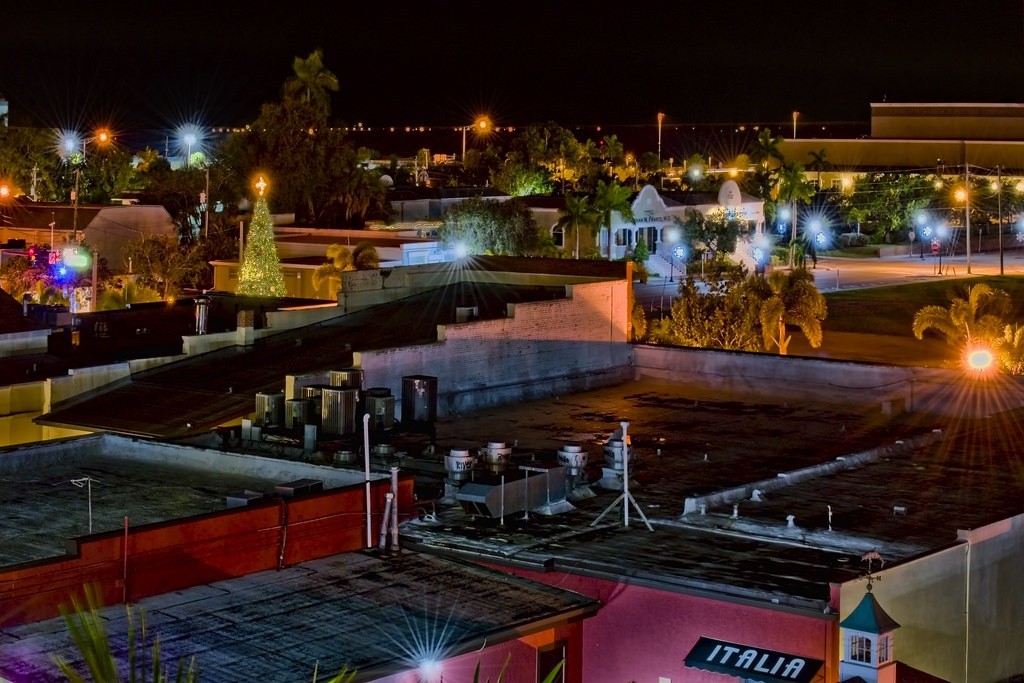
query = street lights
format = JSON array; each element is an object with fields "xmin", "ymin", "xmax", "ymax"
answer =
[
  {"xmin": 991, "ymin": 182, "xmax": 1003, "ymax": 275},
  {"xmin": 64, "ymin": 130, "xmax": 109, "ymax": 164},
  {"xmin": 463, "ymin": 117, "xmax": 487, "ymax": 160},
  {"xmin": 811, "ymin": 220, "xmax": 820, "ymax": 246},
  {"xmin": 955, "ymin": 189, "xmax": 971, "ymax": 274},
  {"xmin": 668, "ymin": 230, "xmax": 679, "ymax": 282},
  {"xmin": 935, "ymin": 225, "xmax": 947, "ymax": 274},
  {"xmin": 917, "ymin": 213, "xmax": 926, "ymax": 258},
  {"xmin": 183, "ymin": 131, "xmax": 197, "ymax": 167}
]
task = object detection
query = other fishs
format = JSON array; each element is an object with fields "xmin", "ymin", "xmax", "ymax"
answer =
[{"xmin": 861, "ymin": 547, "xmax": 887, "ymax": 569}]
